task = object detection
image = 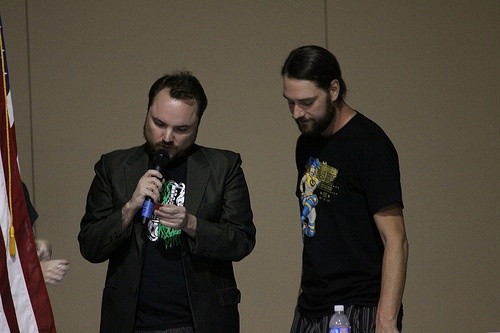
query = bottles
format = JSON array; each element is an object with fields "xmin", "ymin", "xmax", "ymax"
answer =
[{"xmin": 328, "ymin": 305, "xmax": 351, "ymax": 333}]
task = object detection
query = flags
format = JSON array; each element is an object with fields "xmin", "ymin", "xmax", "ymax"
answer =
[{"xmin": 0, "ymin": 21, "xmax": 58, "ymax": 333}]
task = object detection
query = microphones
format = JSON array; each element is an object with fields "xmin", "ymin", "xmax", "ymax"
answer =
[{"xmin": 140, "ymin": 149, "xmax": 170, "ymax": 226}]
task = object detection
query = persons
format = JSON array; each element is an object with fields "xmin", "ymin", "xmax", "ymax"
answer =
[
  {"xmin": 77, "ymin": 68, "xmax": 256, "ymax": 333},
  {"xmin": 278, "ymin": 42, "xmax": 410, "ymax": 333},
  {"xmin": 21, "ymin": 182, "xmax": 70, "ymax": 285}
]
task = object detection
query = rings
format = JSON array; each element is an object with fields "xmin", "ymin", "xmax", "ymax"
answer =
[{"xmin": 57, "ymin": 264, "xmax": 62, "ymax": 269}]
